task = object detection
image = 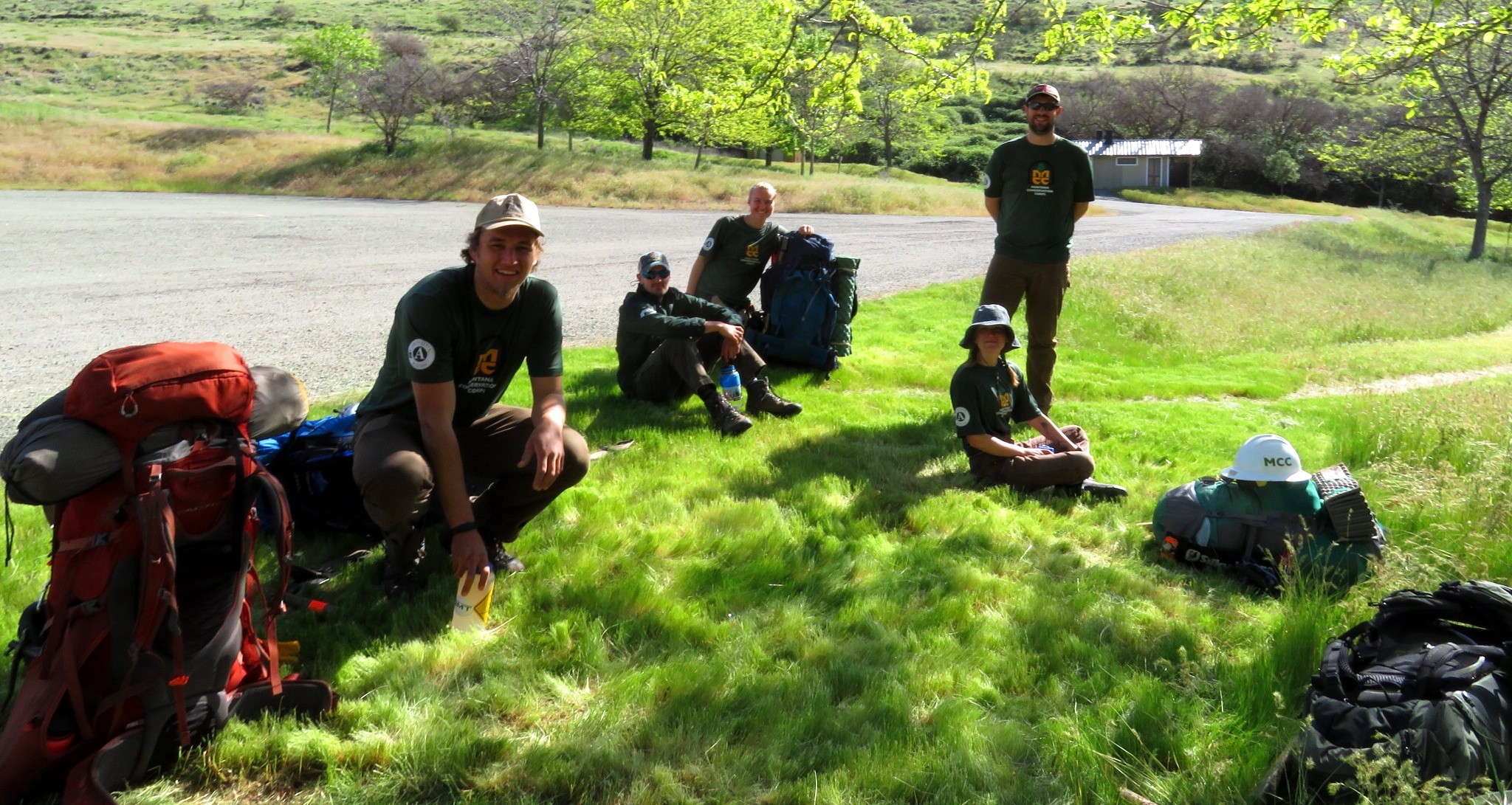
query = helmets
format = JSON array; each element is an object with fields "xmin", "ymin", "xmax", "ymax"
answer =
[{"xmin": 1220, "ymin": 434, "xmax": 1312, "ymax": 482}]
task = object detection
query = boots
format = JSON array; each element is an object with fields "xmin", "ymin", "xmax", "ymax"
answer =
[
  {"xmin": 746, "ymin": 376, "xmax": 802, "ymax": 418},
  {"xmin": 697, "ymin": 384, "xmax": 753, "ymax": 437}
]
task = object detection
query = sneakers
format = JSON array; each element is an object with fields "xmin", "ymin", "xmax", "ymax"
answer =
[
  {"xmin": 385, "ymin": 557, "xmax": 415, "ymax": 603},
  {"xmin": 486, "ymin": 536, "xmax": 524, "ymax": 574}
]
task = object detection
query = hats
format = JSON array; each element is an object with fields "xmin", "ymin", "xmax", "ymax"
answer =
[
  {"xmin": 959, "ymin": 304, "xmax": 1021, "ymax": 353},
  {"xmin": 474, "ymin": 194, "xmax": 545, "ymax": 237},
  {"xmin": 637, "ymin": 251, "xmax": 670, "ymax": 274},
  {"xmin": 1025, "ymin": 84, "xmax": 1060, "ymax": 104}
]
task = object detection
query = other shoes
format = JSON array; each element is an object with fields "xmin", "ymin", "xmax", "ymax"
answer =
[
  {"xmin": 1054, "ymin": 482, "xmax": 1106, "ymax": 506},
  {"xmin": 1080, "ymin": 480, "xmax": 1128, "ymax": 498}
]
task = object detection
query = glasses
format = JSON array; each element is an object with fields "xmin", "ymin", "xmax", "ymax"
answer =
[
  {"xmin": 1026, "ymin": 102, "xmax": 1061, "ymax": 111},
  {"xmin": 642, "ymin": 269, "xmax": 670, "ymax": 280}
]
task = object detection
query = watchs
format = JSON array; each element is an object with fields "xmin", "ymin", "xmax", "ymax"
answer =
[{"xmin": 1074, "ymin": 444, "xmax": 1082, "ymax": 453}]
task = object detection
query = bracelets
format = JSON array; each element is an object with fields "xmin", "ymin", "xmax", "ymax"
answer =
[{"xmin": 447, "ymin": 520, "xmax": 481, "ymax": 536}]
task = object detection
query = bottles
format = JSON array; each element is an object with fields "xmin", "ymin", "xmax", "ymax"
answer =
[
  {"xmin": 720, "ymin": 365, "xmax": 741, "ymax": 401},
  {"xmin": 451, "ymin": 562, "xmax": 495, "ymax": 634},
  {"xmin": 1039, "ymin": 446, "xmax": 1054, "ymax": 454}
]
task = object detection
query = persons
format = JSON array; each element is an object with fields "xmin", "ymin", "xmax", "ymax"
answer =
[
  {"xmin": 950, "ymin": 304, "xmax": 1093, "ymax": 500},
  {"xmin": 686, "ymin": 182, "xmax": 814, "ymax": 333},
  {"xmin": 616, "ymin": 252, "xmax": 804, "ymax": 435},
  {"xmin": 968, "ymin": 84, "xmax": 1095, "ymax": 417},
  {"xmin": 353, "ymin": 193, "xmax": 591, "ymax": 596}
]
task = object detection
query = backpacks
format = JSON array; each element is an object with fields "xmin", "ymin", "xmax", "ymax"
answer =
[
  {"xmin": 0, "ymin": 342, "xmax": 342, "ymax": 805},
  {"xmin": 1251, "ymin": 579, "xmax": 1512, "ymax": 805},
  {"xmin": 254, "ymin": 402, "xmax": 379, "ymax": 538},
  {"xmin": 1152, "ymin": 463, "xmax": 1387, "ymax": 596},
  {"xmin": 761, "ymin": 231, "xmax": 859, "ymax": 381}
]
